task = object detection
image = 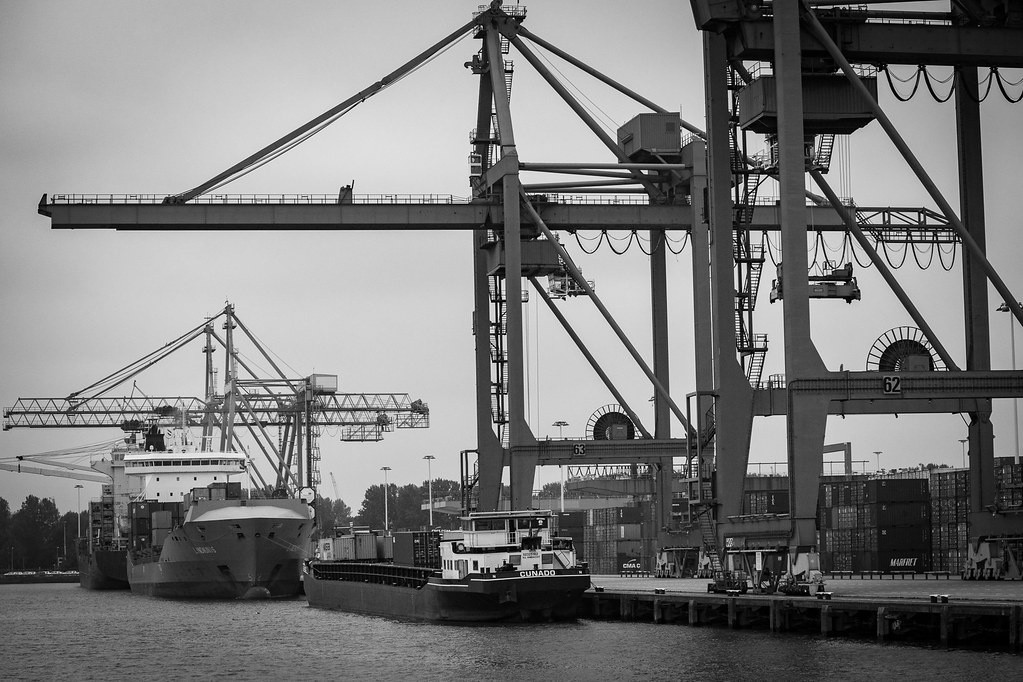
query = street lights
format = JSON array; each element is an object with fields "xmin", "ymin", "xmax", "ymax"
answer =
[
  {"xmin": 553, "ymin": 420, "xmax": 570, "ymax": 511},
  {"xmin": 422, "ymin": 455, "xmax": 436, "ymax": 528},
  {"xmin": 379, "ymin": 467, "xmax": 392, "ymax": 529},
  {"xmin": 997, "ymin": 302, "xmax": 1023, "ymax": 465},
  {"xmin": 74, "ymin": 484, "xmax": 85, "ymax": 537}
]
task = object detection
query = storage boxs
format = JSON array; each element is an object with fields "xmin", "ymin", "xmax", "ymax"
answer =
[
  {"xmin": 124, "ymin": 481, "xmax": 242, "ymax": 556},
  {"xmin": 317, "ymin": 531, "xmax": 441, "ymax": 566}
]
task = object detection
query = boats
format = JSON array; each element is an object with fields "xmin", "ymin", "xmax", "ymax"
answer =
[{"xmin": 303, "ymin": 508, "xmax": 590, "ymax": 625}]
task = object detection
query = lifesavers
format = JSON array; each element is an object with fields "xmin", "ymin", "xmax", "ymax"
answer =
[
  {"xmin": 147, "ymin": 443, "xmax": 156, "ymax": 452},
  {"xmin": 336, "ymin": 531, "xmax": 344, "ymax": 538},
  {"xmin": 456, "ymin": 543, "xmax": 466, "ymax": 552}
]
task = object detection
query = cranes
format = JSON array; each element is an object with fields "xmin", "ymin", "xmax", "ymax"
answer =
[
  {"xmin": 37, "ymin": 0, "xmax": 948, "ymax": 506},
  {"xmin": 2, "ymin": 299, "xmax": 432, "ymax": 528}
]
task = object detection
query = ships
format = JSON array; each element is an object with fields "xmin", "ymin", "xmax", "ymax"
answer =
[{"xmin": 72, "ymin": 405, "xmax": 313, "ymax": 601}]
